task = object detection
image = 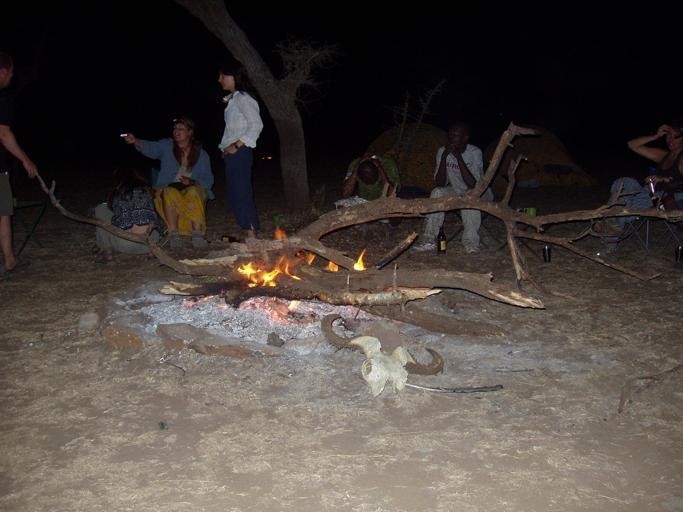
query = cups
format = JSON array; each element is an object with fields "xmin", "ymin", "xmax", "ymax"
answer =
[
  {"xmin": 675, "ymin": 245, "xmax": 683, "ymax": 261},
  {"xmin": 524, "ymin": 207, "xmax": 536, "ymax": 218},
  {"xmin": 543, "ymin": 245, "xmax": 552, "ymax": 262}
]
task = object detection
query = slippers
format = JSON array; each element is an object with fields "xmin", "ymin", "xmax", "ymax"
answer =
[{"xmin": 4, "ymin": 259, "xmax": 30, "ymax": 276}]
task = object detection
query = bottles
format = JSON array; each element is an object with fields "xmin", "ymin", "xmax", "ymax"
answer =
[
  {"xmin": 437, "ymin": 226, "xmax": 446, "ymax": 255},
  {"xmin": 650, "ymin": 192, "xmax": 665, "ymax": 212},
  {"xmin": 221, "ymin": 235, "xmax": 242, "ymax": 243},
  {"xmin": 275, "ymin": 226, "xmax": 288, "ymax": 241}
]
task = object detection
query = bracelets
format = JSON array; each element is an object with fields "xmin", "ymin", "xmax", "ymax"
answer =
[
  {"xmin": 375, "ymin": 164, "xmax": 381, "ymax": 169},
  {"xmin": 191, "ymin": 179, "xmax": 194, "ymax": 186}
]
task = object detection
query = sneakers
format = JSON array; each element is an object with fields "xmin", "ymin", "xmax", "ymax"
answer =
[
  {"xmin": 413, "ymin": 242, "xmax": 437, "ymax": 252},
  {"xmin": 464, "ymin": 241, "xmax": 480, "ymax": 254}
]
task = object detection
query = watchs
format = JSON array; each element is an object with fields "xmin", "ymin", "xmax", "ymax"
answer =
[{"xmin": 234, "ymin": 143, "xmax": 240, "ymax": 150}]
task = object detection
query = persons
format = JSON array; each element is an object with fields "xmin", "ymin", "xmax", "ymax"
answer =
[
  {"xmin": 333, "ymin": 141, "xmax": 401, "ymax": 243},
  {"xmin": 217, "ymin": 61, "xmax": 264, "ymax": 230},
  {"xmin": 0, "ymin": 50, "xmax": 38, "ymax": 272},
  {"xmin": 93, "ymin": 165, "xmax": 164, "ymax": 263},
  {"xmin": 120, "ymin": 118, "xmax": 216, "ymax": 236},
  {"xmin": 413, "ymin": 122, "xmax": 494, "ymax": 255},
  {"xmin": 569, "ymin": 124, "xmax": 683, "ymax": 253}
]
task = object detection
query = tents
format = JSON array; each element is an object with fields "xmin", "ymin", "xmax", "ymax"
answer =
[
  {"xmin": 483, "ymin": 124, "xmax": 594, "ymax": 190},
  {"xmin": 364, "ymin": 122, "xmax": 449, "ymax": 197}
]
